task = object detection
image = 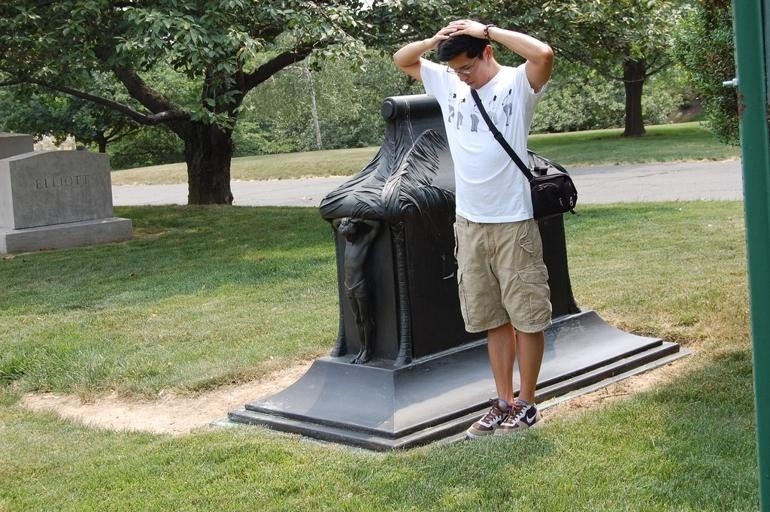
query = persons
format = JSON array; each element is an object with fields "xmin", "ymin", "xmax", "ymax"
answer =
[
  {"xmin": 331, "ymin": 217, "xmax": 380, "ymax": 366},
  {"xmin": 390, "ymin": 15, "xmax": 555, "ymax": 441}
]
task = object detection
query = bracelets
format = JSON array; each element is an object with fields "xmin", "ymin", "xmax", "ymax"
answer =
[{"xmin": 482, "ymin": 23, "xmax": 497, "ymax": 42}]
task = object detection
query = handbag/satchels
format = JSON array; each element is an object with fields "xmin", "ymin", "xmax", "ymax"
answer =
[{"xmin": 527, "ymin": 149, "xmax": 578, "ymax": 219}]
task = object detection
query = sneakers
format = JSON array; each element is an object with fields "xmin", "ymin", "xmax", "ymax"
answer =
[
  {"xmin": 465, "ymin": 399, "xmax": 514, "ymax": 440},
  {"xmin": 493, "ymin": 399, "xmax": 545, "ymax": 436}
]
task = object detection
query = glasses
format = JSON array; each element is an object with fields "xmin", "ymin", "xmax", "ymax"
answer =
[{"xmin": 447, "ymin": 55, "xmax": 480, "ymax": 75}]
